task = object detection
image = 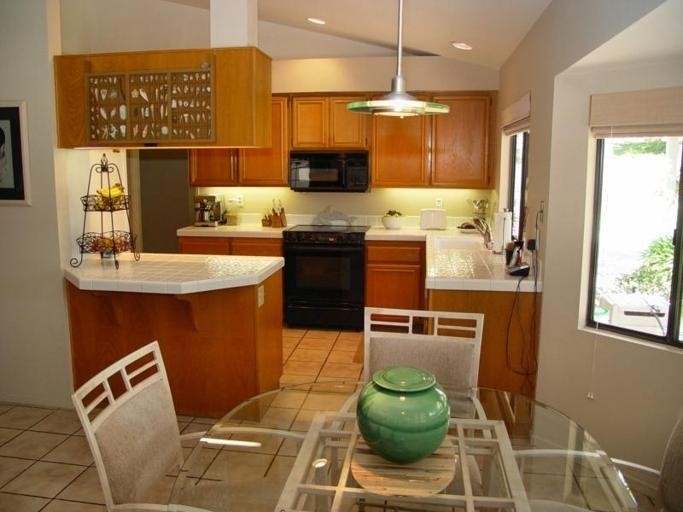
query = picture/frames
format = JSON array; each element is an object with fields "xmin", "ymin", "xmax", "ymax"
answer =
[{"xmin": 0, "ymin": 100, "xmax": 32, "ymax": 208}]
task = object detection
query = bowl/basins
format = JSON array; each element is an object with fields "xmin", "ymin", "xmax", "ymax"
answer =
[{"xmin": 381, "ymin": 216, "xmax": 403, "ymax": 229}]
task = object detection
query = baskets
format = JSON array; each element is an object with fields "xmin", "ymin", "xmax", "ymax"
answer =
[
  {"xmin": 76, "ymin": 230, "xmax": 137, "ymax": 255},
  {"xmin": 80, "ymin": 195, "xmax": 130, "ymax": 211}
]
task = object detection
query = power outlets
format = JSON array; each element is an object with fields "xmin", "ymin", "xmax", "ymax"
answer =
[
  {"xmin": 533, "ymin": 229, "xmax": 539, "ymax": 251},
  {"xmin": 540, "ymin": 201, "xmax": 544, "ymax": 223}
]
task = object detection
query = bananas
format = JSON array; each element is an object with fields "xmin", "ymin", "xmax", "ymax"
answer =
[{"xmin": 94, "ymin": 183, "xmax": 125, "ymax": 211}]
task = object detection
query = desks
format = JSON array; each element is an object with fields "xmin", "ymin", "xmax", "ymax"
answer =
[{"xmin": 168, "ymin": 381, "xmax": 639, "ymax": 512}]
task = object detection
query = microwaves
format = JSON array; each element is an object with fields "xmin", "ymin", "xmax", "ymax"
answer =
[{"xmin": 287, "ymin": 147, "xmax": 369, "ymax": 193}]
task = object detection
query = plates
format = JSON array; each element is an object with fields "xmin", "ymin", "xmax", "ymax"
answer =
[{"xmin": 456, "ymin": 225, "xmax": 477, "ymax": 232}]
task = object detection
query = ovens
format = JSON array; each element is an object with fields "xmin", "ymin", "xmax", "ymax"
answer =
[{"xmin": 282, "ymin": 232, "xmax": 364, "ymax": 330}]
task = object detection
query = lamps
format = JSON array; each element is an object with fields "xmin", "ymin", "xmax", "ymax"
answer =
[{"xmin": 346, "ymin": 0, "xmax": 450, "ymax": 118}]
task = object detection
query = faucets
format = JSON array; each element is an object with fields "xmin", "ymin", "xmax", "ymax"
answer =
[{"xmin": 462, "ymin": 218, "xmax": 490, "ymax": 246}]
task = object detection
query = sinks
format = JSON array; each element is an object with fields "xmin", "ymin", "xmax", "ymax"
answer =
[{"xmin": 435, "ymin": 236, "xmax": 485, "ymax": 250}]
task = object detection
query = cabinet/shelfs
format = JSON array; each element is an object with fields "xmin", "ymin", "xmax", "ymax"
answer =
[
  {"xmin": 179, "ymin": 236, "xmax": 282, "ymax": 256},
  {"xmin": 53, "ymin": 45, "xmax": 272, "ymax": 148},
  {"xmin": 191, "ymin": 92, "xmax": 288, "ymax": 186},
  {"xmin": 365, "ymin": 239, "xmax": 427, "ymax": 326},
  {"xmin": 289, "ymin": 91, "xmax": 371, "ymax": 151},
  {"xmin": 369, "ymin": 88, "xmax": 497, "ymax": 189}
]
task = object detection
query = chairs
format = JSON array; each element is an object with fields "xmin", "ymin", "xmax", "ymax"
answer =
[
  {"xmin": 528, "ymin": 418, "xmax": 683, "ymax": 512},
  {"xmin": 72, "ymin": 339, "xmax": 279, "ymax": 512},
  {"xmin": 364, "ymin": 307, "xmax": 485, "ymax": 494}
]
